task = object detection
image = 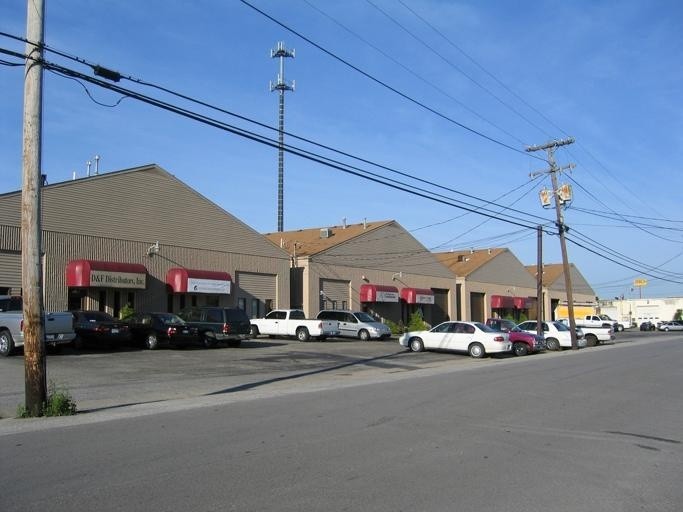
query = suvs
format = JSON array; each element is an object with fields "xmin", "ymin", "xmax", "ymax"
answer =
[{"xmin": 175, "ymin": 305, "xmax": 250, "ymax": 349}]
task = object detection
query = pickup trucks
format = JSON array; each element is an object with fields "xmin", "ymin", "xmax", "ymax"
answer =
[
  {"xmin": 554, "ymin": 318, "xmax": 614, "ymax": 347},
  {"xmin": 574, "ymin": 315, "xmax": 618, "ymax": 333},
  {"xmin": 0, "ymin": 294, "xmax": 76, "ymax": 357},
  {"xmin": 454, "ymin": 318, "xmax": 545, "ymax": 356},
  {"xmin": 248, "ymin": 309, "xmax": 340, "ymax": 342}
]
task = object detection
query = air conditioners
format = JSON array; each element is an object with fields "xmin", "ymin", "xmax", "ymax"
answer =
[{"xmin": 319, "ymin": 228, "xmax": 330, "ymax": 238}]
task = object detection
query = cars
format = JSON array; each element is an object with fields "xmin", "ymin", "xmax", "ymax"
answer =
[
  {"xmin": 639, "ymin": 322, "xmax": 654, "ymax": 331},
  {"xmin": 596, "ymin": 314, "xmax": 631, "ymax": 332},
  {"xmin": 62, "ymin": 308, "xmax": 129, "ymax": 352},
  {"xmin": 657, "ymin": 322, "xmax": 682, "ymax": 332},
  {"xmin": 398, "ymin": 320, "xmax": 510, "ymax": 360},
  {"xmin": 120, "ymin": 311, "xmax": 193, "ymax": 350},
  {"xmin": 500, "ymin": 320, "xmax": 586, "ymax": 352}
]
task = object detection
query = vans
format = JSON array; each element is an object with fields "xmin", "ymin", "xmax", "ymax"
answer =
[{"xmin": 315, "ymin": 310, "xmax": 391, "ymax": 341}]
